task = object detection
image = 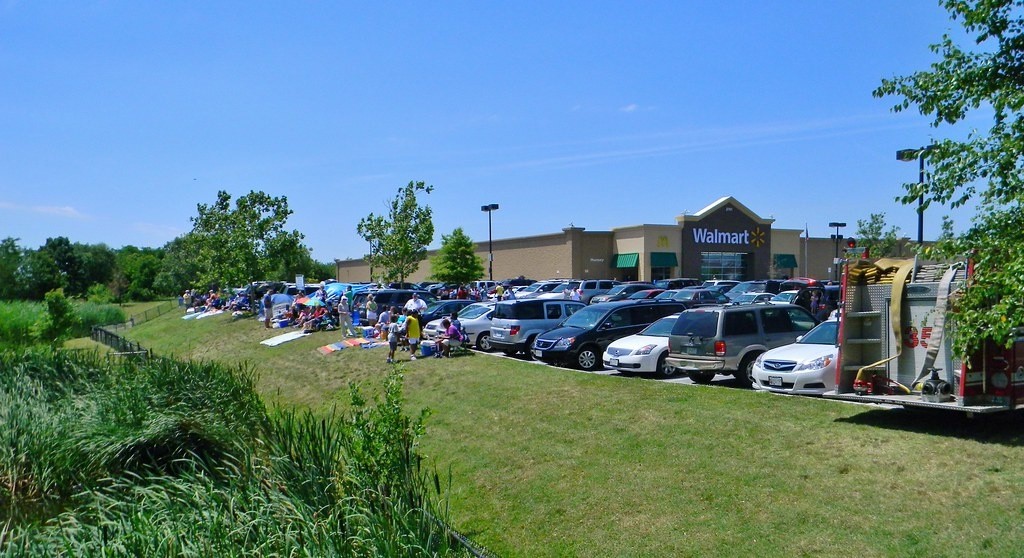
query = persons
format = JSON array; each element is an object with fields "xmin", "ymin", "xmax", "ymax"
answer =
[
  {"xmin": 827, "ymin": 281, "xmax": 832, "ymax": 285},
  {"xmin": 449, "ymin": 312, "xmax": 461, "ymax": 333},
  {"xmin": 436, "ymin": 281, "xmax": 516, "ymax": 301},
  {"xmin": 182, "ymin": 283, "xmax": 428, "ymax": 363},
  {"xmin": 435, "ymin": 319, "xmax": 464, "ymax": 356},
  {"xmin": 810, "ymin": 291, "xmax": 818, "ymax": 315},
  {"xmin": 562, "ymin": 286, "xmax": 586, "ymax": 302}
]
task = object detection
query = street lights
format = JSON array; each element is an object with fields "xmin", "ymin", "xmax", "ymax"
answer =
[
  {"xmin": 480, "ymin": 203, "xmax": 500, "ymax": 282},
  {"xmin": 829, "ymin": 223, "xmax": 845, "ymax": 280},
  {"xmin": 896, "ymin": 144, "xmax": 935, "ymax": 243}
]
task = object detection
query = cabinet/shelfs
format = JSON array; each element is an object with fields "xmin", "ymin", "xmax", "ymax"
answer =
[{"xmin": 843, "ymin": 310, "xmax": 886, "ymax": 371}]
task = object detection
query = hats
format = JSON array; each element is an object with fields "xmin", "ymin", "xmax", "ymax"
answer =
[{"xmin": 341, "ymin": 296, "xmax": 348, "ymax": 301}]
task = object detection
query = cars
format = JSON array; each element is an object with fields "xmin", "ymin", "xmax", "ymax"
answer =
[
  {"xmin": 440, "ymin": 275, "xmax": 842, "ymax": 304},
  {"xmin": 225, "ymin": 279, "xmax": 440, "ymax": 327},
  {"xmin": 418, "ymin": 299, "xmax": 477, "ymax": 325},
  {"xmin": 602, "ymin": 313, "xmax": 681, "ymax": 379},
  {"xmin": 421, "ymin": 302, "xmax": 494, "ymax": 353},
  {"xmin": 752, "ymin": 317, "xmax": 840, "ymax": 396}
]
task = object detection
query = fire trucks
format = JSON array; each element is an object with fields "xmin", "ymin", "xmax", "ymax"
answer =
[{"xmin": 823, "ymin": 244, "xmax": 1024, "ymax": 413}]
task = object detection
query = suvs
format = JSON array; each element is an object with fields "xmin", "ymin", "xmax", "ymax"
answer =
[
  {"xmin": 529, "ymin": 298, "xmax": 686, "ymax": 371},
  {"xmin": 486, "ymin": 298, "xmax": 588, "ymax": 357},
  {"xmin": 664, "ymin": 302, "xmax": 821, "ymax": 390}
]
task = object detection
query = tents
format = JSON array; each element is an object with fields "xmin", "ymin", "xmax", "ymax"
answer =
[{"xmin": 258, "ymin": 293, "xmax": 295, "ymax": 319}]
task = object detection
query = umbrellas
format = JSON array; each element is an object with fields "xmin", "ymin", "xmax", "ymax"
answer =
[
  {"xmin": 296, "ymin": 297, "xmax": 311, "ymax": 304},
  {"xmin": 304, "ymin": 297, "xmax": 326, "ymax": 306}
]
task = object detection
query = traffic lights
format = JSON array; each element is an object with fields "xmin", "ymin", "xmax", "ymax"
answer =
[{"xmin": 847, "ymin": 237, "xmax": 856, "ymax": 247}]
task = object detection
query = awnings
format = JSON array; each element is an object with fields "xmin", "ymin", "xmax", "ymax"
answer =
[
  {"xmin": 773, "ymin": 253, "xmax": 798, "ymax": 270},
  {"xmin": 651, "ymin": 251, "xmax": 679, "ymax": 267},
  {"xmin": 609, "ymin": 252, "xmax": 639, "ymax": 268}
]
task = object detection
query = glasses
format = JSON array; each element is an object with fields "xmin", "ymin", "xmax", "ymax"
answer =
[{"xmin": 343, "ymin": 299, "xmax": 347, "ymax": 301}]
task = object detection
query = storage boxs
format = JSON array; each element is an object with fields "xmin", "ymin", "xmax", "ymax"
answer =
[
  {"xmin": 363, "ymin": 325, "xmax": 375, "ymax": 337},
  {"xmin": 419, "ymin": 340, "xmax": 436, "ymax": 356},
  {"xmin": 279, "ymin": 318, "xmax": 288, "ymax": 328}
]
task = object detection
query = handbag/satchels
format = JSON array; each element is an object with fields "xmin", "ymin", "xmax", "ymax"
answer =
[
  {"xmin": 461, "ymin": 331, "xmax": 470, "ymax": 343},
  {"xmin": 304, "ymin": 330, "xmax": 312, "ymax": 334},
  {"xmin": 433, "ymin": 352, "xmax": 440, "ymax": 358}
]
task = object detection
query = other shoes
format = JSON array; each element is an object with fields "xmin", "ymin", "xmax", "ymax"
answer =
[
  {"xmin": 387, "ymin": 359, "xmax": 391, "ymax": 362},
  {"xmin": 411, "ymin": 354, "xmax": 417, "ymax": 361},
  {"xmin": 391, "ymin": 359, "xmax": 397, "ymax": 363},
  {"xmin": 354, "ymin": 334, "xmax": 359, "ymax": 337},
  {"xmin": 400, "ymin": 347, "xmax": 404, "ymax": 351},
  {"xmin": 405, "ymin": 348, "xmax": 409, "ymax": 352},
  {"xmin": 441, "ymin": 351, "xmax": 449, "ymax": 357},
  {"xmin": 343, "ymin": 337, "xmax": 346, "ymax": 339}
]
